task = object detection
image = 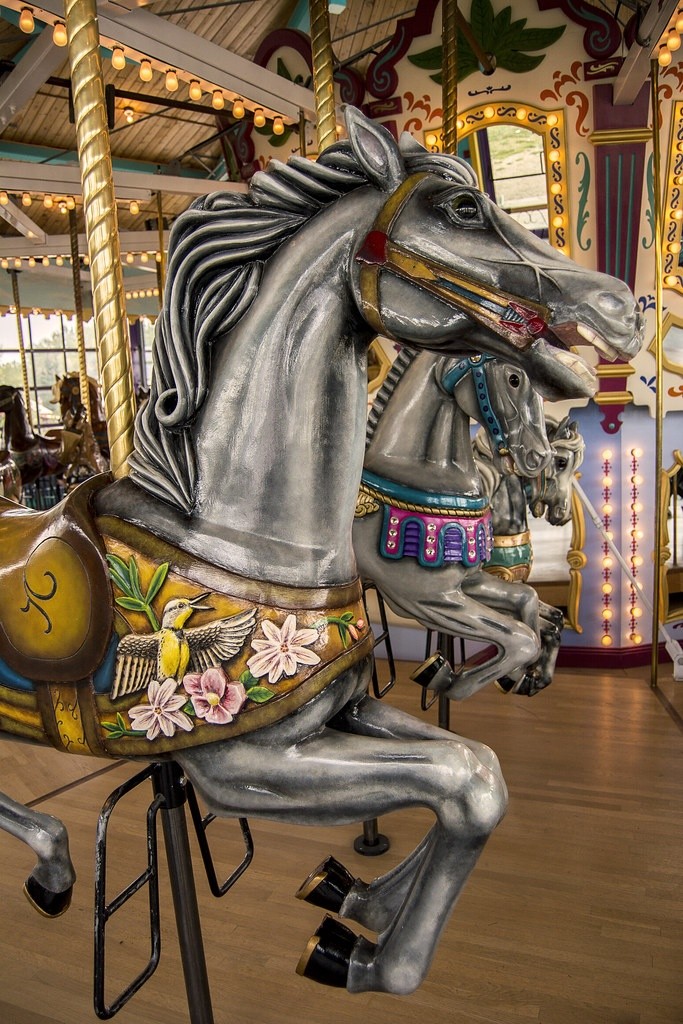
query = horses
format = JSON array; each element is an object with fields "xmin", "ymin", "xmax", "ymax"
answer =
[{"xmin": 0, "ymin": 102, "xmax": 644, "ymax": 993}]
